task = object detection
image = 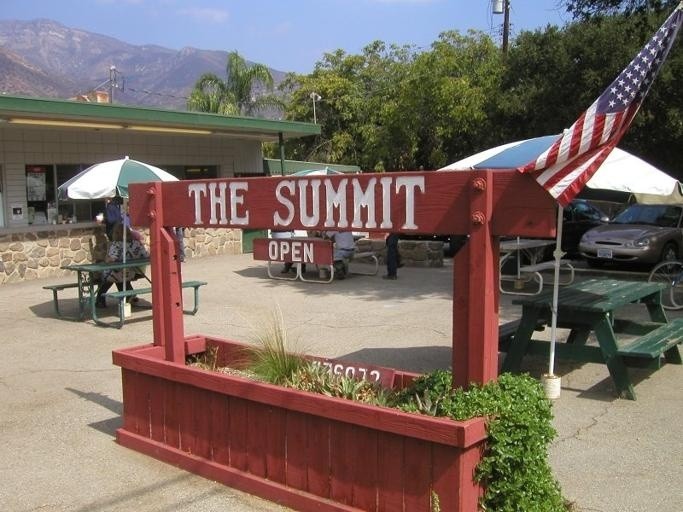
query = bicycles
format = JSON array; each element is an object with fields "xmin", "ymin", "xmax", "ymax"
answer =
[{"xmin": 647, "ymin": 259, "xmax": 683, "ymax": 310}]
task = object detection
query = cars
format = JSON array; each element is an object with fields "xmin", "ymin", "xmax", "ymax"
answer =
[
  {"xmin": 562, "ymin": 199, "xmax": 612, "ymax": 259},
  {"xmin": 578, "ymin": 203, "xmax": 683, "ymax": 265}
]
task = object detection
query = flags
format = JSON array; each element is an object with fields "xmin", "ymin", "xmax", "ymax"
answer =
[{"xmin": 517, "ymin": 0, "xmax": 683, "ymax": 209}]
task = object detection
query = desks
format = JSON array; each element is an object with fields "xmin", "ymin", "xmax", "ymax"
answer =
[
  {"xmin": 499, "ymin": 277, "xmax": 668, "ymax": 400},
  {"xmin": 292, "ymin": 235, "xmax": 365, "ymax": 242},
  {"xmin": 499, "ymin": 238, "xmax": 559, "ymax": 250},
  {"xmin": 60, "ymin": 254, "xmax": 163, "ymax": 271}
]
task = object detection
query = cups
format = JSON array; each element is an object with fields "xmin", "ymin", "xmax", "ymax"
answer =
[
  {"xmin": 62, "ymin": 220, "xmax": 66, "ymax": 224},
  {"xmin": 69, "ymin": 218, "xmax": 73, "ymax": 223},
  {"xmin": 52, "ymin": 219, "xmax": 57, "ymax": 225}
]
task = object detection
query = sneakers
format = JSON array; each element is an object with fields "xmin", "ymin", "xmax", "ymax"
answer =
[
  {"xmin": 131, "ymin": 296, "xmax": 139, "ymax": 302},
  {"xmin": 95, "ymin": 302, "xmax": 107, "ymax": 307},
  {"xmin": 396, "ymin": 256, "xmax": 403, "ymax": 267},
  {"xmin": 281, "ymin": 264, "xmax": 292, "ymax": 273},
  {"xmin": 383, "ymin": 274, "xmax": 396, "ymax": 280},
  {"xmin": 293, "ymin": 267, "xmax": 306, "ymax": 273}
]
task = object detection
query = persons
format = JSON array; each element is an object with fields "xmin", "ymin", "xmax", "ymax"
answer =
[
  {"xmin": 322, "ymin": 229, "xmax": 355, "ymax": 280},
  {"xmin": 105, "ymin": 196, "xmax": 122, "ymax": 240},
  {"xmin": 89, "ymin": 223, "xmax": 146, "ymax": 308},
  {"xmin": 171, "ymin": 226, "xmax": 186, "ymax": 262},
  {"xmin": 280, "ymin": 261, "xmax": 306, "ymax": 273},
  {"xmin": 386, "ymin": 233, "xmax": 402, "ymax": 280}
]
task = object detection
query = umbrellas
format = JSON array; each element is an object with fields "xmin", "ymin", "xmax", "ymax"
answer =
[
  {"xmin": 289, "ymin": 165, "xmax": 348, "ymax": 175},
  {"xmin": 434, "ymin": 127, "xmax": 683, "ymax": 399},
  {"xmin": 58, "ymin": 155, "xmax": 180, "ymax": 318}
]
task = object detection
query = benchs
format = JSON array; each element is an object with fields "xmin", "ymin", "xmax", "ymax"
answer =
[
  {"xmin": 266, "ymin": 249, "xmax": 299, "ymax": 281},
  {"xmin": 616, "ymin": 318, "xmax": 683, "ymax": 370},
  {"xmin": 42, "ymin": 273, "xmax": 142, "ymax": 323},
  {"xmin": 496, "ymin": 311, "xmax": 552, "ymax": 353},
  {"xmin": 92, "ymin": 281, "xmax": 207, "ymax": 330},
  {"xmin": 300, "ymin": 245, "xmax": 379, "ymax": 284},
  {"xmin": 520, "ymin": 259, "xmax": 575, "ymax": 297}
]
